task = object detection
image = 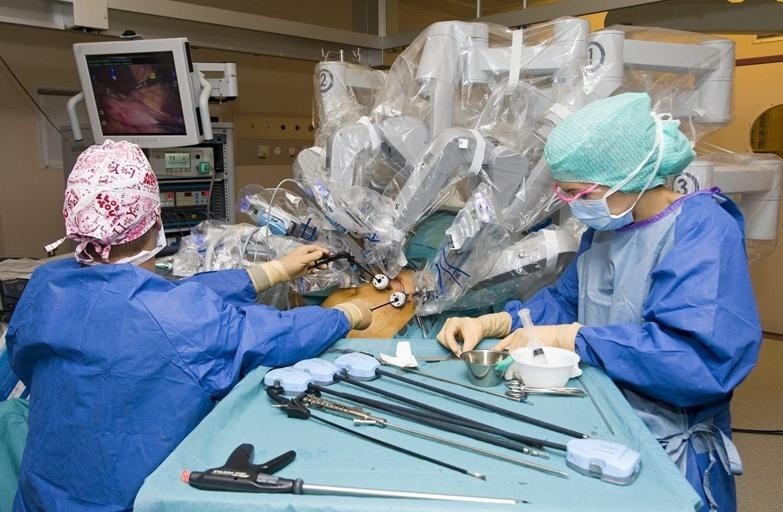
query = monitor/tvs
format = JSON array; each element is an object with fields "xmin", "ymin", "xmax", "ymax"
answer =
[{"xmin": 73, "ymin": 37, "xmax": 204, "ymax": 149}]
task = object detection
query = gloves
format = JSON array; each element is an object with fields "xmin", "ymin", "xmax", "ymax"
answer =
[
  {"xmin": 492, "ymin": 321, "xmax": 585, "ymax": 353},
  {"xmin": 335, "ymin": 298, "xmax": 373, "ymax": 330},
  {"xmin": 246, "ymin": 244, "xmax": 330, "ymax": 294},
  {"xmin": 436, "ymin": 311, "xmax": 513, "ymax": 359}
]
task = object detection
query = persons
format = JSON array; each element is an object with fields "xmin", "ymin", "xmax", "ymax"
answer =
[
  {"xmin": 435, "ymin": 91, "xmax": 764, "ymax": 511},
  {"xmin": 319, "ymin": 269, "xmax": 430, "ymax": 340},
  {"xmin": 3, "ymin": 139, "xmax": 373, "ymax": 511}
]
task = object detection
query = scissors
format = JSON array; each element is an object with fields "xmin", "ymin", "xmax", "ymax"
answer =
[{"xmin": 506, "ymin": 380, "xmax": 588, "ymax": 398}]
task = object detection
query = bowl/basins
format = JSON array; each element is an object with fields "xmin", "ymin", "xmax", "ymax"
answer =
[
  {"xmin": 461, "ymin": 349, "xmax": 512, "ymax": 387},
  {"xmin": 509, "ymin": 347, "xmax": 580, "ymax": 389}
]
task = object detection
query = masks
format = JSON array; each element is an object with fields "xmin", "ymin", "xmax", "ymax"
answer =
[{"xmin": 568, "ymin": 110, "xmax": 674, "ymax": 233}]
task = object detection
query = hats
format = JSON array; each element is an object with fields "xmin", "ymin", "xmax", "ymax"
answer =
[
  {"xmin": 43, "ymin": 140, "xmax": 162, "ymax": 266},
  {"xmin": 543, "ymin": 90, "xmax": 697, "ymax": 194}
]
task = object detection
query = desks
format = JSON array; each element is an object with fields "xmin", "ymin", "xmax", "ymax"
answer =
[{"xmin": 133, "ymin": 338, "xmax": 702, "ymax": 512}]
